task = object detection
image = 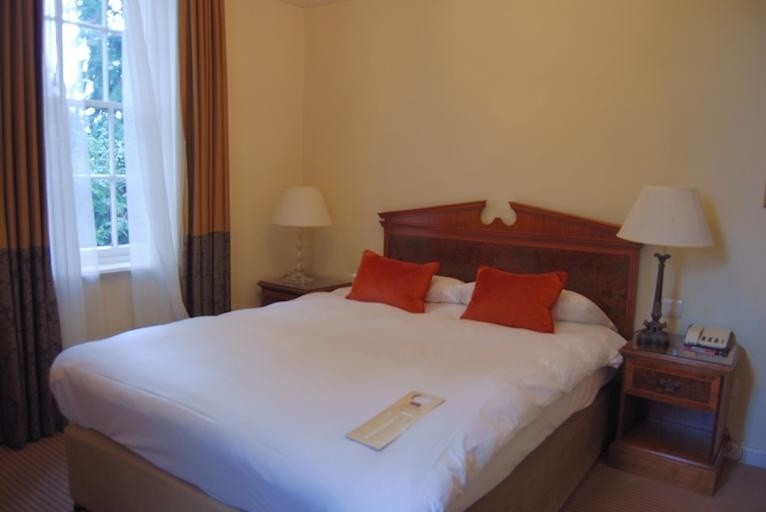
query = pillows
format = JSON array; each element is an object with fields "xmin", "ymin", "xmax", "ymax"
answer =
[{"xmin": 347, "ymin": 247, "xmax": 619, "ymax": 336}]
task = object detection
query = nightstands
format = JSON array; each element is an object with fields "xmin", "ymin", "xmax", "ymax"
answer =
[
  {"xmin": 257, "ymin": 278, "xmax": 352, "ymax": 306},
  {"xmin": 608, "ymin": 335, "xmax": 745, "ymax": 498}
]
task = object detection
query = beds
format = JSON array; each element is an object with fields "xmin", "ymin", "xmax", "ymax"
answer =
[{"xmin": 48, "ymin": 199, "xmax": 644, "ymax": 512}]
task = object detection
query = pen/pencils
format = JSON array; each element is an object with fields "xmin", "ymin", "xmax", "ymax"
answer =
[{"xmin": 685, "ymin": 346, "xmax": 727, "ymax": 357}]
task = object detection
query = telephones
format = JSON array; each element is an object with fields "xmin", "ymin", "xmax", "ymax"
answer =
[{"xmin": 685, "ymin": 322, "xmax": 732, "ymax": 350}]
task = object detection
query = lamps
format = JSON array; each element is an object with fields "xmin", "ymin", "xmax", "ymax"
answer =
[
  {"xmin": 618, "ymin": 186, "xmax": 715, "ymax": 350},
  {"xmin": 272, "ymin": 186, "xmax": 332, "ymax": 283}
]
